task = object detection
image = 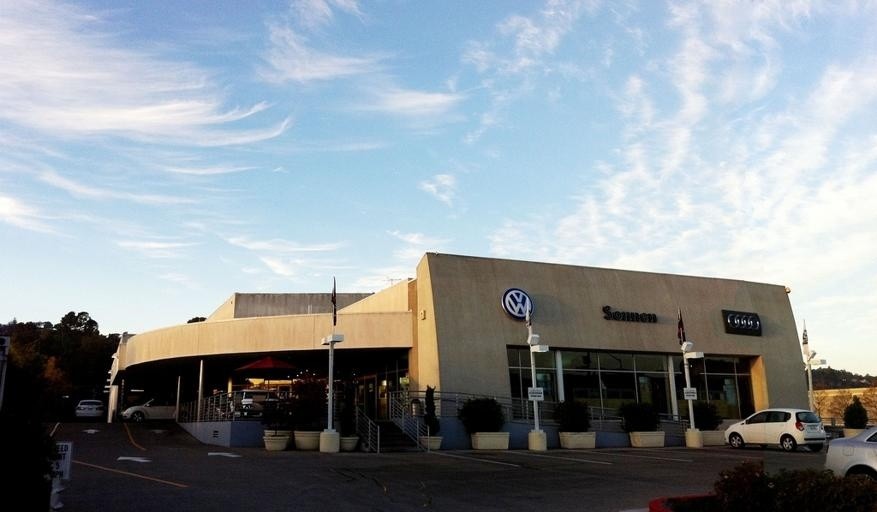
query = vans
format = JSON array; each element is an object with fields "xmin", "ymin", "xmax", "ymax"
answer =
[{"xmin": 720, "ymin": 407, "xmax": 827, "ymax": 450}]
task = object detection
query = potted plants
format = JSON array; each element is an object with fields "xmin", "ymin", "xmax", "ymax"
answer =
[
  {"xmin": 263, "ymin": 377, "xmax": 361, "ymax": 451},
  {"xmin": 457, "ymin": 397, "xmax": 511, "ymax": 450},
  {"xmin": 842, "ymin": 393, "xmax": 869, "ymax": 436},
  {"xmin": 616, "ymin": 401, "xmax": 666, "ymax": 448},
  {"xmin": 687, "ymin": 403, "xmax": 725, "ymax": 446},
  {"xmin": 418, "ymin": 384, "xmax": 442, "ymax": 450},
  {"xmin": 551, "ymin": 399, "xmax": 596, "ymax": 449}
]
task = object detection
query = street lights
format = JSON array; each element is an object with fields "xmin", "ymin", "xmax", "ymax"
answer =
[
  {"xmin": 805, "ymin": 348, "xmax": 826, "ymax": 412},
  {"xmin": 525, "ymin": 330, "xmax": 548, "ymax": 450},
  {"xmin": 317, "ymin": 332, "xmax": 343, "ymax": 453},
  {"xmin": 681, "ymin": 338, "xmax": 704, "ymax": 451}
]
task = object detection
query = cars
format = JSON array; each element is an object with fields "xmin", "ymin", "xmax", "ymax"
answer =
[
  {"xmin": 232, "ymin": 388, "xmax": 282, "ymax": 416},
  {"xmin": 75, "ymin": 399, "xmax": 105, "ymax": 418},
  {"xmin": 823, "ymin": 426, "xmax": 876, "ymax": 481},
  {"xmin": 122, "ymin": 395, "xmax": 178, "ymax": 422}
]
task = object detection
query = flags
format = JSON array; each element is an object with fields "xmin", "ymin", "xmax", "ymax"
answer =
[
  {"xmin": 801, "ymin": 322, "xmax": 809, "ymax": 358},
  {"xmin": 331, "ymin": 276, "xmax": 338, "ymax": 327},
  {"xmin": 675, "ymin": 307, "xmax": 687, "ymax": 345}
]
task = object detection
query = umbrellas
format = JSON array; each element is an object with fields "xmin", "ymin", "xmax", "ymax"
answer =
[{"xmin": 230, "ymin": 354, "xmax": 296, "ymax": 399}]
